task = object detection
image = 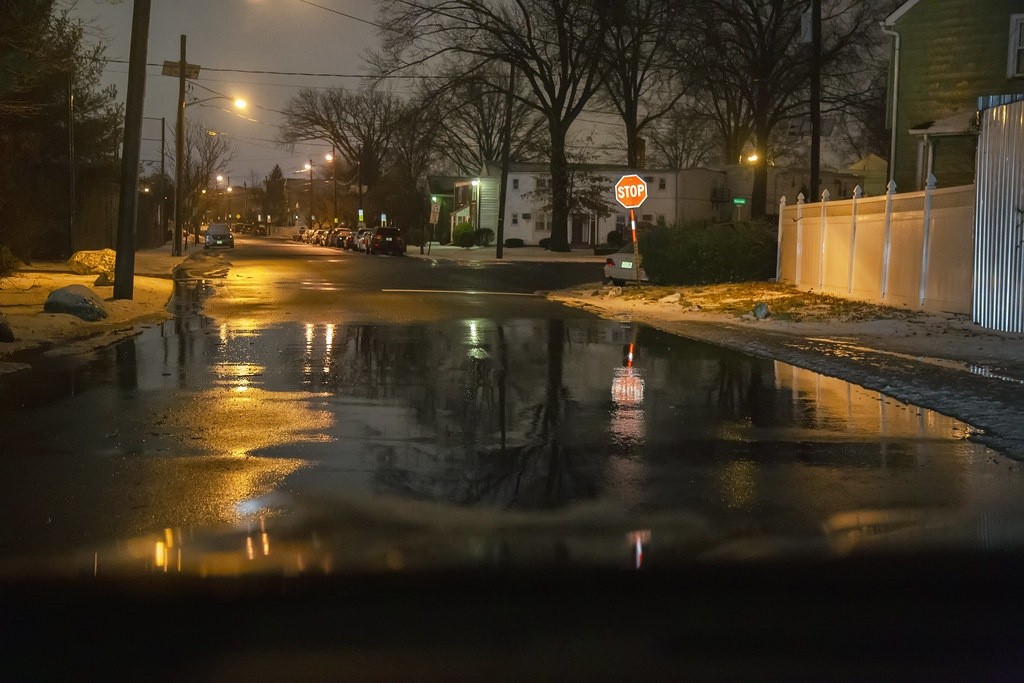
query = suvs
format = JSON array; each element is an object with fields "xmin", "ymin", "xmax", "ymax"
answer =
[{"xmin": 370, "ymin": 226, "xmax": 406, "ymax": 256}]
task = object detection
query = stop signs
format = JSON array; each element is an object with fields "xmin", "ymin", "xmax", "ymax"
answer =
[{"xmin": 615, "ymin": 174, "xmax": 648, "ymax": 209}]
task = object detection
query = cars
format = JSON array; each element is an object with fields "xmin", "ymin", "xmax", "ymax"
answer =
[
  {"xmin": 201, "ymin": 223, "xmax": 235, "ymax": 249},
  {"xmin": 301, "ymin": 228, "xmax": 374, "ymax": 255},
  {"xmin": 231, "ymin": 221, "xmax": 267, "ymax": 236},
  {"xmin": 292, "ymin": 229, "xmax": 305, "ymax": 242}
]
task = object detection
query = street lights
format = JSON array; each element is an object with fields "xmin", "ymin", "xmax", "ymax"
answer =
[
  {"xmin": 171, "ymin": 94, "xmax": 249, "ymax": 254},
  {"xmin": 304, "ymin": 163, "xmax": 314, "ymax": 230},
  {"xmin": 326, "ymin": 155, "xmax": 339, "ymax": 228},
  {"xmin": 215, "ymin": 174, "xmax": 223, "ymax": 222}
]
